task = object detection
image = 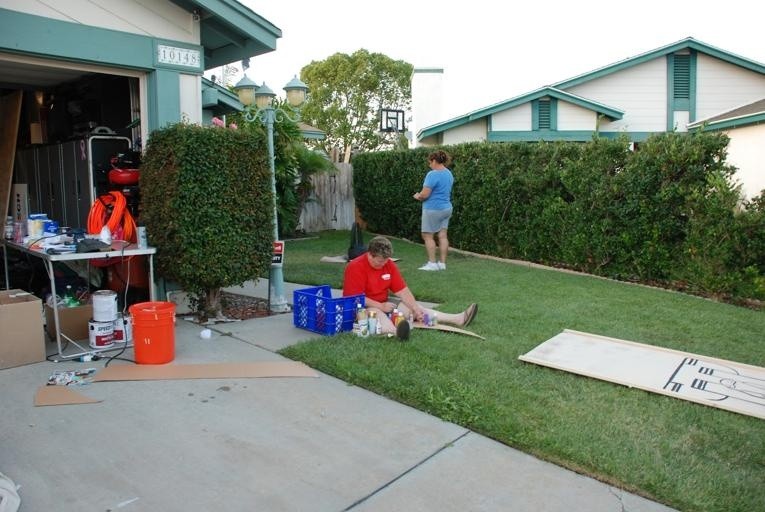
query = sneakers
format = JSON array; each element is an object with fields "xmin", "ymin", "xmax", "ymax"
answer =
[
  {"xmin": 438, "ymin": 261, "xmax": 447, "ymax": 271},
  {"xmin": 419, "ymin": 262, "xmax": 440, "ymax": 272}
]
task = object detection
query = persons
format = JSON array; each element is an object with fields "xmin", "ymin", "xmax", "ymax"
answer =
[
  {"xmin": 342, "ymin": 237, "xmax": 479, "ymax": 342},
  {"xmin": 412, "ymin": 150, "xmax": 455, "ymax": 273}
]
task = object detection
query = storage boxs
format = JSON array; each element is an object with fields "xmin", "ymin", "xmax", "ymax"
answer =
[
  {"xmin": 42, "ymin": 302, "xmax": 95, "ymax": 343},
  {"xmin": 1, "ymin": 287, "xmax": 49, "ymax": 371}
]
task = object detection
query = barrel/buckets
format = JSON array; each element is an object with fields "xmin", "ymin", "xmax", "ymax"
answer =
[
  {"xmin": 92, "ymin": 290, "xmax": 117, "ymax": 322},
  {"xmin": 129, "ymin": 302, "xmax": 175, "ymax": 365},
  {"xmin": 112, "ymin": 313, "xmax": 133, "ymax": 342},
  {"xmin": 87, "ymin": 321, "xmax": 114, "ymax": 349}
]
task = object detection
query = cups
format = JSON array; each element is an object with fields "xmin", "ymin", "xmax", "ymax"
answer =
[{"xmin": 14, "ymin": 222, "xmax": 25, "ymax": 245}]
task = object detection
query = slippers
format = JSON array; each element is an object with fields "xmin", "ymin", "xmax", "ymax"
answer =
[
  {"xmin": 462, "ymin": 301, "xmax": 478, "ymax": 328},
  {"xmin": 394, "ymin": 319, "xmax": 411, "ymax": 341}
]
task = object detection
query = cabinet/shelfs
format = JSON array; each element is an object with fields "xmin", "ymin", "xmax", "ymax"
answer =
[{"xmin": 8, "ymin": 132, "xmax": 132, "ymax": 230}]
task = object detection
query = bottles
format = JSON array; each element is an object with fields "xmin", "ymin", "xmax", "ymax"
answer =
[
  {"xmin": 356, "ymin": 302, "xmax": 382, "ymax": 337},
  {"xmin": 5, "ymin": 216, "xmax": 15, "ymax": 240},
  {"xmin": 63, "ymin": 284, "xmax": 82, "ymax": 308},
  {"xmin": 391, "ymin": 306, "xmax": 438, "ymax": 331}
]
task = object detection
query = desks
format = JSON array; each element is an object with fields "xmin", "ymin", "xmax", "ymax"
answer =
[{"xmin": 1, "ymin": 225, "xmax": 157, "ymax": 358}]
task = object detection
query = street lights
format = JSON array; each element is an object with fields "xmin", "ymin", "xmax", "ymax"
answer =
[{"xmin": 236, "ymin": 73, "xmax": 311, "ymax": 312}]
